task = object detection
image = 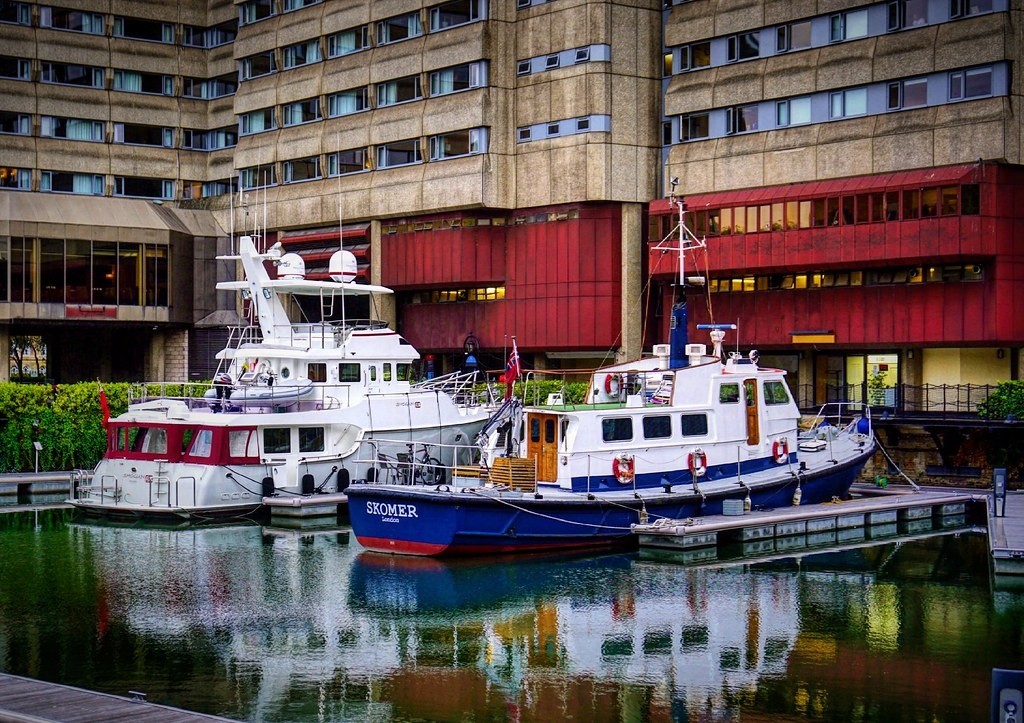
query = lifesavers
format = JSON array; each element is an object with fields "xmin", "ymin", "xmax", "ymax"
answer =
[
  {"xmin": 613, "ymin": 452, "xmax": 634, "ymax": 484},
  {"xmin": 605, "ymin": 372, "xmax": 624, "ymax": 398},
  {"xmin": 688, "ymin": 447, "xmax": 707, "ymax": 477}
]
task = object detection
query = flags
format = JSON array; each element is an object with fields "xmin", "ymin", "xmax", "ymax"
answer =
[{"xmin": 500, "ymin": 345, "xmax": 522, "ymax": 384}]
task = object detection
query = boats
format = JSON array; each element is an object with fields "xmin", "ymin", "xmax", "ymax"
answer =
[
  {"xmin": 345, "ymin": 152, "xmax": 881, "ymax": 556},
  {"xmin": 60, "ymin": 132, "xmax": 520, "ymax": 527}
]
